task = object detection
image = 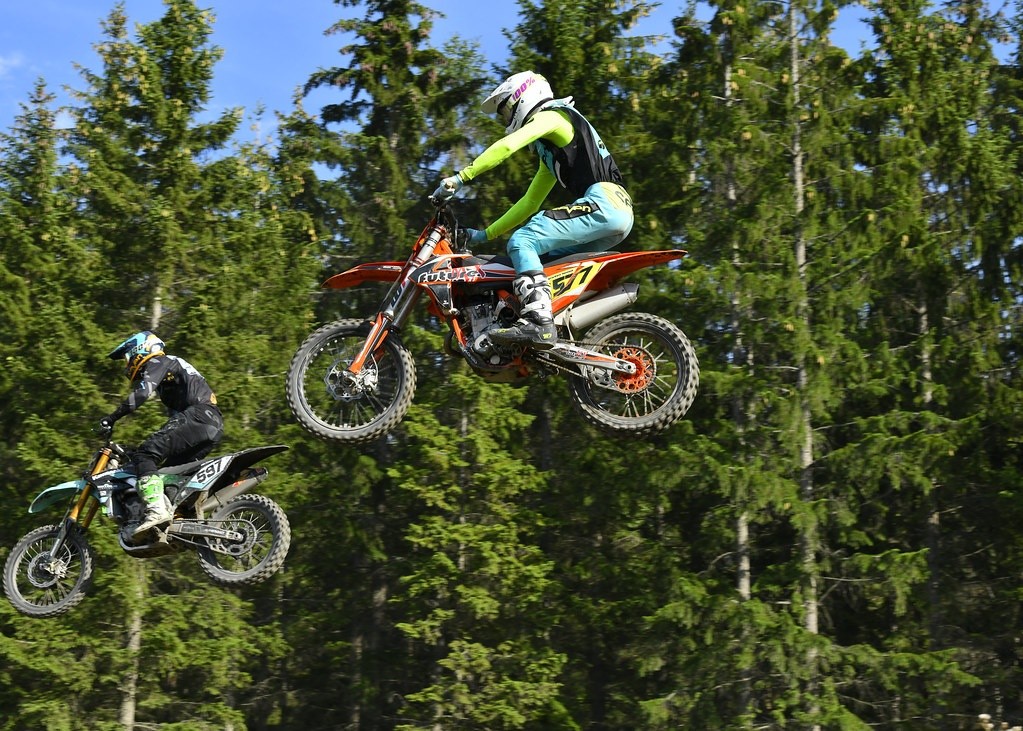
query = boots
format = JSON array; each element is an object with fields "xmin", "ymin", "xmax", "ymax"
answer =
[
  {"xmin": 129, "ymin": 472, "xmax": 174, "ymax": 540},
  {"xmin": 487, "ymin": 270, "xmax": 559, "ymax": 350}
]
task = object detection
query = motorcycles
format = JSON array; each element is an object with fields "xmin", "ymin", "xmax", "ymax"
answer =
[
  {"xmin": 2, "ymin": 421, "xmax": 291, "ymax": 619},
  {"xmin": 285, "ymin": 180, "xmax": 702, "ymax": 444}
]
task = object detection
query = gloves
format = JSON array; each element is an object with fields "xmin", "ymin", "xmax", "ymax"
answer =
[
  {"xmin": 98, "ymin": 416, "xmax": 114, "ymax": 439},
  {"xmin": 434, "ymin": 174, "xmax": 463, "ymax": 200},
  {"xmin": 458, "ymin": 228, "xmax": 489, "ymax": 247}
]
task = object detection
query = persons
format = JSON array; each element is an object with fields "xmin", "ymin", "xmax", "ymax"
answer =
[
  {"xmin": 431, "ymin": 68, "xmax": 635, "ymax": 349},
  {"xmin": 96, "ymin": 332, "xmax": 224, "ymax": 538}
]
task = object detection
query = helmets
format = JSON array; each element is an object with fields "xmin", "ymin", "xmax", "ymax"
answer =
[
  {"xmin": 480, "ymin": 70, "xmax": 554, "ymax": 135},
  {"xmin": 105, "ymin": 331, "xmax": 165, "ymax": 381}
]
